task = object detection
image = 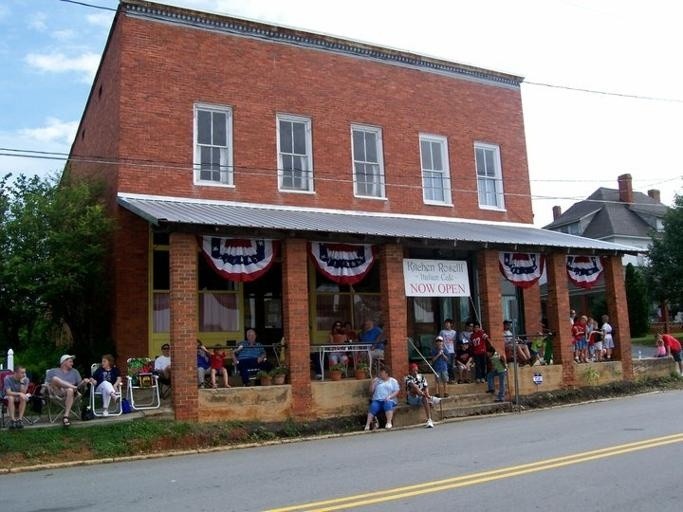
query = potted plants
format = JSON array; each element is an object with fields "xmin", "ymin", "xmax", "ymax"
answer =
[
  {"xmin": 256, "ymin": 371, "xmax": 272, "ymax": 386},
  {"xmin": 268, "ymin": 367, "xmax": 290, "ymax": 384},
  {"xmin": 355, "ymin": 363, "xmax": 369, "ymax": 380},
  {"xmin": 329, "ymin": 363, "xmax": 347, "ymax": 381}
]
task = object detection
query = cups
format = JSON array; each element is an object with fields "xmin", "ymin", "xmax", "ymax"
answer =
[{"xmin": 143, "ymin": 368, "xmax": 147, "ymax": 372}]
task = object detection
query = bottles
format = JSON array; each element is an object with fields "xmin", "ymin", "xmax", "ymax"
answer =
[{"xmin": 637, "ymin": 348, "xmax": 641, "ymax": 358}]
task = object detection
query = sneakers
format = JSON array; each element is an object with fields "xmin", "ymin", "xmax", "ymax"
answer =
[
  {"xmin": 114, "ymin": 396, "xmax": 120, "ymax": 404},
  {"xmin": 434, "ymin": 393, "xmax": 450, "ymax": 398},
  {"xmin": 7, "ymin": 420, "xmax": 25, "ymax": 429},
  {"xmin": 485, "ymin": 388, "xmax": 506, "ymax": 404},
  {"xmin": 103, "ymin": 410, "xmax": 108, "ymax": 418},
  {"xmin": 426, "ymin": 419, "xmax": 433, "ymax": 428},
  {"xmin": 433, "ymin": 396, "xmax": 441, "ymax": 405}
]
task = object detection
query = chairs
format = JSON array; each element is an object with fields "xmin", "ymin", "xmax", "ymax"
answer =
[
  {"xmin": 88, "ymin": 363, "xmax": 123, "ymax": 417},
  {"xmin": 0, "ymin": 370, "xmax": 41, "ymax": 428},
  {"xmin": 41, "ymin": 370, "xmax": 82, "ymax": 423},
  {"xmin": 163, "ymin": 358, "xmax": 233, "ymax": 400},
  {"xmin": 126, "ymin": 358, "xmax": 161, "ymax": 410}
]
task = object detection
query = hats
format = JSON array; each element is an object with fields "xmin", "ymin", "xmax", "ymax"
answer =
[
  {"xmin": 408, "ymin": 335, "xmax": 446, "ymax": 371},
  {"xmin": 59, "ymin": 354, "xmax": 75, "ymax": 363}
]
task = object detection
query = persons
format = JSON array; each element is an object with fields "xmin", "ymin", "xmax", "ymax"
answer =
[
  {"xmin": 405, "ymin": 363, "xmax": 440, "ymax": 428},
  {"xmin": 431, "ymin": 310, "xmax": 615, "ymax": 403},
  {"xmin": 153, "ymin": 329, "xmax": 267, "ymax": 387},
  {"xmin": 41, "ymin": 353, "xmax": 89, "ymax": 427},
  {"xmin": 364, "ymin": 366, "xmax": 401, "ymax": 431},
  {"xmin": 655, "ymin": 339, "xmax": 666, "ymax": 357},
  {"xmin": 655, "ymin": 332, "xmax": 683, "ymax": 373},
  {"xmin": 3, "ymin": 365, "xmax": 29, "ymax": 429},
  {"xmin": 87, "ymin": 353, "xmax": 120, "ymax": 415},
  {"xmin": 328, "ymin": 320, "xmax": 384, "ymax": 363}
]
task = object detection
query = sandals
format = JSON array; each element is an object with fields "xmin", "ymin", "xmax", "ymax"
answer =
[{"xmin": 61, "ymin": 416, "xmax": 71, "ymax": 427}]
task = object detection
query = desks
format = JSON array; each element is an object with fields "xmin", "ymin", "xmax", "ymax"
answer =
[{"xmin": 310, "ymin": 344, "xmax": 373, "ymax": 381}]
